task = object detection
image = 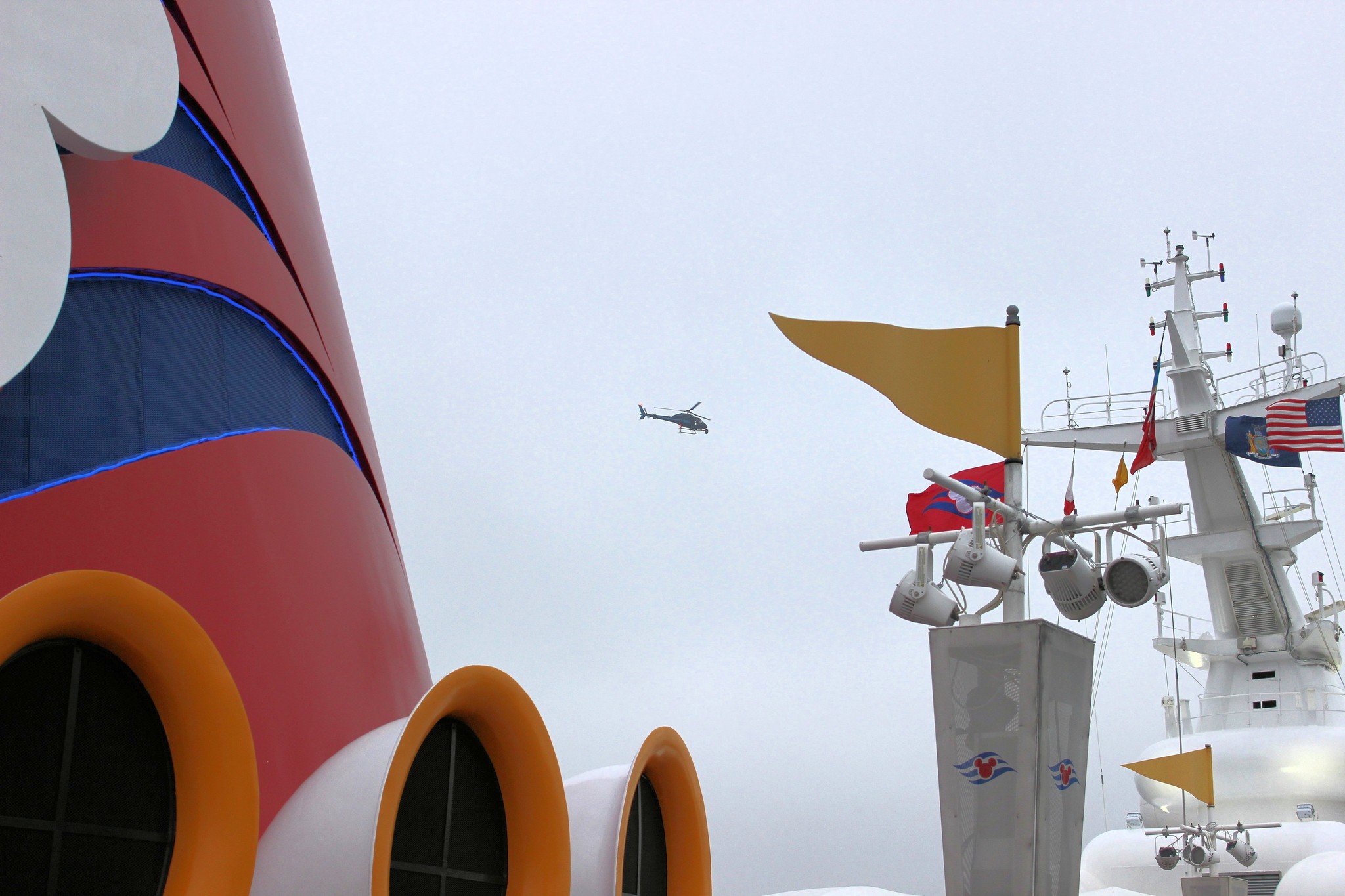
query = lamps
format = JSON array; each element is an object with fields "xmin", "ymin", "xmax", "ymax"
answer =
[
  {"xmin": 1038, "ymin": 527, "xmax": 1106, "ymax": 621},
  {"xmin": 943, "ymin": 502, "xmax": 1026, "ymax": 592},
  {"xmin": 1101, "ymin": 519, "xmax": 1171, "ymax": 610},
  {"xmin": 1155, "ymin": 829, "xmax": 1257, "ymax": 872},
  {"xmin": 888, "ymin": 543, "xmax": 960, "ymax": 626},
  {"xmin": 1126, "ymin": 813, "xmax": 1143, "ymax": 829},
  {"xmin": 1296, "ymin": 804, "xmax": 1316, "ymax": 821}
]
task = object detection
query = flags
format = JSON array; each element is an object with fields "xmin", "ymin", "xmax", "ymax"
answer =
[
  {"xmin": 1264, "ymin": 396, "xmax": 1345, "ymax": 452},
  {"xmin": 1130, "ymin": 316, "xmax": 1167, "ymax": 475},
  {"xmin": 1225, "ymin": 415, "xmax": 1302, "ymax": 468},
  {"xmin": 906, "ymin": 461, "xmax": 1005, "ymax": 536},
  {"xmin": 1064, "ymin": 461, "xmax": 1075, "ymax": 515},
  {"xmin": 1113, "ymin": 455, "xmax": 1127, "ymax": 493}
]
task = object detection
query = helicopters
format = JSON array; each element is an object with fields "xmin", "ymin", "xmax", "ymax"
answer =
[{"xmin": 638, "ymin": 401, "xmax": 711, "ymax": 434}]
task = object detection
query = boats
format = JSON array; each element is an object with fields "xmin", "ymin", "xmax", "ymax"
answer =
[{"xmin": 857, "ymin": 227, "xmax": 1344, "ymax": 896}]
